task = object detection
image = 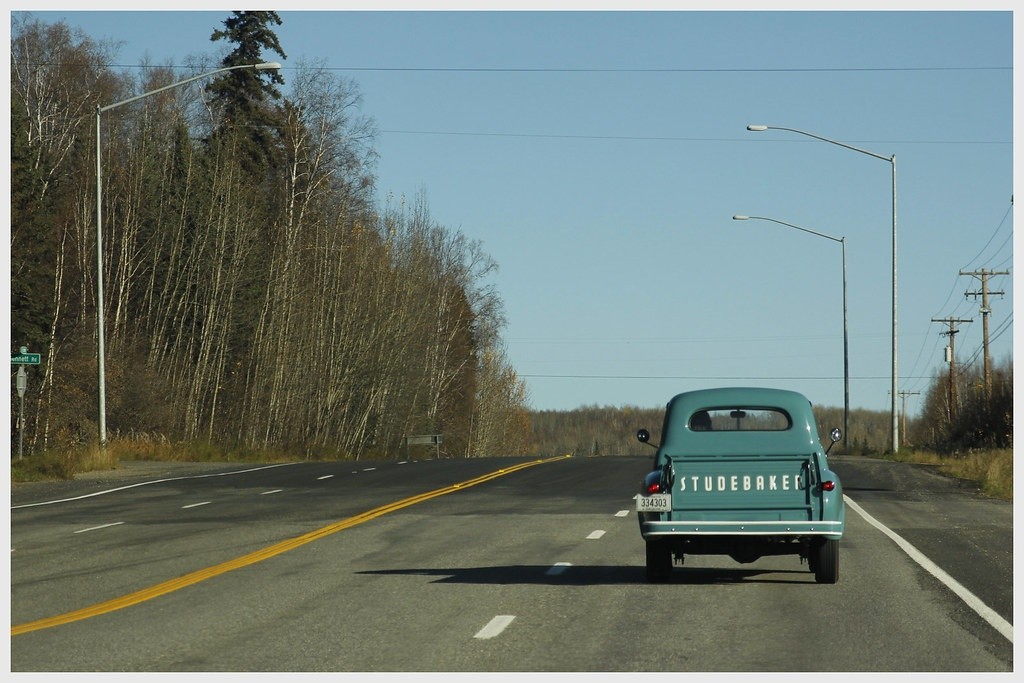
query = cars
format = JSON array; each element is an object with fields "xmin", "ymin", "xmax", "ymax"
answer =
[{"xmin": 635, "ymin": 386, "xmax": 846, "ymax": 584}]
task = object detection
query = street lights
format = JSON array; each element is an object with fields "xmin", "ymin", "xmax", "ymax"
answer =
[
  {"xmin": 95, "ymin": 60, "xmax": 282, "ymax": 447},
  {"xmin": 732, "ymin": 214, "xmax": 852, "ymax": 448},
  {"xmin": 747, "ymin": 123, "xmax": 901, "ymax": 454}
]
